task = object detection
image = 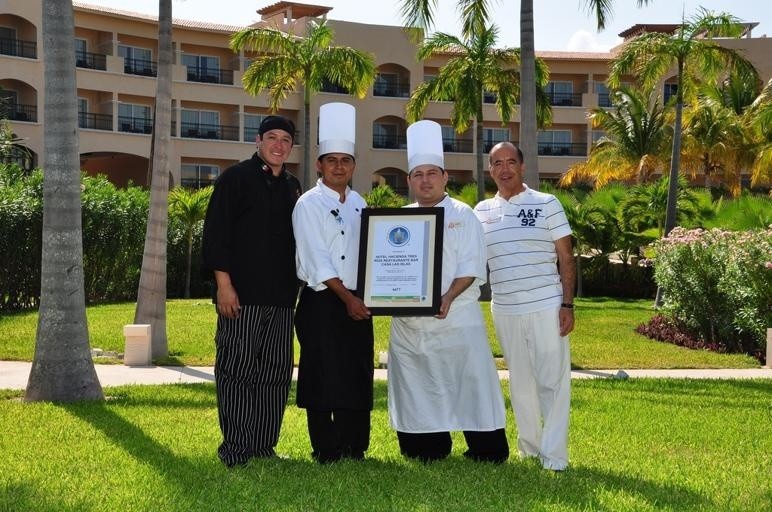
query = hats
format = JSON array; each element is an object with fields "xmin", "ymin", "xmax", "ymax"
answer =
[
  {"xmin": 405, "ymin": 119, "xmax": 445, "ymax": 174},
  {"xmin": 258, "ymin": 116, "xmax": 295, "ymax": 137},
  {"xmin": 316, "ymin": 101, "xmax": 356, "ymax": 161}
]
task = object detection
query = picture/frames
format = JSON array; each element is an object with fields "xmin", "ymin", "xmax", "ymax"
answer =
[{"xmin": 356, "ymin": 207, "xmax": 445, "ymax": 316}]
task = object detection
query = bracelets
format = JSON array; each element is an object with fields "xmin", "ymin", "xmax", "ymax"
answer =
[{"xmin": 560, "ymin": 303, "xmax": 574, "ymax": 309}]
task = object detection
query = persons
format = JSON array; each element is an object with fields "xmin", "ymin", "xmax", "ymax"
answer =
[
  {"xmin": 202, "ymin": 116, "xmax": 302, "ymax": 469},
  {"xmin": 472, "ymin": 142, "xmax": 577, "ymax": 472},
  {"xmin": 291, "ymin": 152, "xmax": 373, "ymax": 465},
  {"xmin": 387, "ymin": 164, "xmax": 510, "ymax": 466}
]
